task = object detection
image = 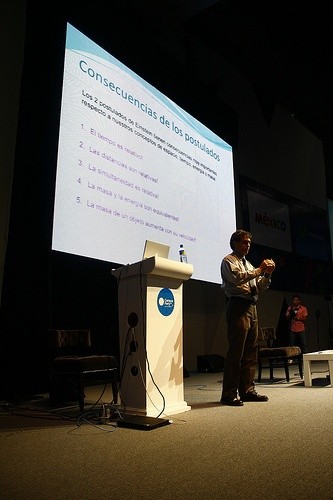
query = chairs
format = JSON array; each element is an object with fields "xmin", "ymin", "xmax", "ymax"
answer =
[
  {"xmin": 49, "ymin": 329, "xmax": 118, "ymax": 411},
  {"xmin": 257, "ymin": 327, "xmax": 303, "ymax": 383}
]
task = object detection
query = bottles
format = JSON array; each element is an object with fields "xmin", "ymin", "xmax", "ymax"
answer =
[{"xmin": 179, "ymin": 245, "xmax": 187, "ymax": 262}]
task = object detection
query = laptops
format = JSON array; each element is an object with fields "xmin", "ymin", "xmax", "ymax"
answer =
[
  {"xmin": 117, "ymin": 415, "xmax": 169, "ymax": 431},
  {"xmin": 142, "ymin": 240, "xmax": 170, "ymax": 260}
]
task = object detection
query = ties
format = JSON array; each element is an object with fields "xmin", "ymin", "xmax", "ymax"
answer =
[{"xmin": 242, "ymin": 258, "xmax": 257, "ymax": 296}]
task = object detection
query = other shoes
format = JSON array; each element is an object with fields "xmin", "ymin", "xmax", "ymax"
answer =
[
  {"xmin": 243, "ymin": 391, "xmax": 269, "ymax": 402},
  {"xmin": 220, "ymin": 396, "xmax": 244, "ymax": 406}
]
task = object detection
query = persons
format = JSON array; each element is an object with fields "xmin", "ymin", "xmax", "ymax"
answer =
[
  {"xmin": 285, "ymin": 296, "xmax": 308, "ymax": 365},
  {"xmin": 219, "ymin": 230, "xmax": 275, "ymax": 407}
]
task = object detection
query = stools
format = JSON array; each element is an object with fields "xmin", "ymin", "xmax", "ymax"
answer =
[{"xmin": 302, "ymin": 349, "xmax": 333, "ymax": 387}]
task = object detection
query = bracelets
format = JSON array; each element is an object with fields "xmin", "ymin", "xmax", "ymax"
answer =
[{"xmin": 264, "ymin": 272, "xmax": 272, "ymax": 278}]
